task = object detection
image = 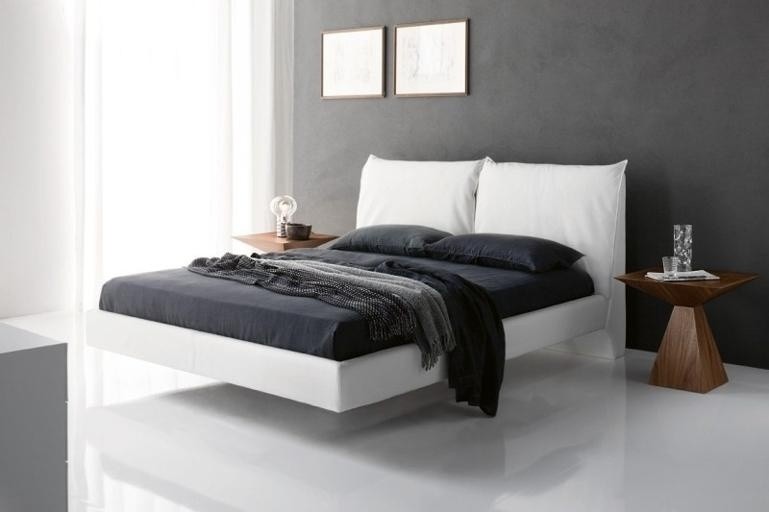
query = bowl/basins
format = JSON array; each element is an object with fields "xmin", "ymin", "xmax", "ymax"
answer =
[{"xmin": 284, "ymin": 223, "xmax": 311, "ymax": 239}]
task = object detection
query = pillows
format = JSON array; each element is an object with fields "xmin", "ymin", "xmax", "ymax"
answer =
[{"xmin": 330, "ymin": 150, "xmax": 629, "ymax": 267}]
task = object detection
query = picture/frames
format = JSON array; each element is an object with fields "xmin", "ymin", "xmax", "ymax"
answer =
[
  {"xmin": 319, "ymin": 25, "xmax": 387, "ymax": 101},
  {"xmin": 393, "ymin": 17, "xmax": 470, "ymax": 97}
]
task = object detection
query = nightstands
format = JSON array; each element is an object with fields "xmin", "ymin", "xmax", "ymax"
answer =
[
  {"xmin": 615, "ymin": 257, "xmax": 759, "ymax": 397},
  {"xmin": 235, "ymin": 230, "xmax": 343, "ymax": 254}
]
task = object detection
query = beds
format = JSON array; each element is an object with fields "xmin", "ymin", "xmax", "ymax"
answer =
[{"xmin": 94, "ymin": 152, "xmax": 625, "ymax": 415}]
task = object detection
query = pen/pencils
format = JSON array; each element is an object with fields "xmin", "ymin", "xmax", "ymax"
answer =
[{"xmin": 678, "ymin": 276, "xmax": 705, "ymax": 278}]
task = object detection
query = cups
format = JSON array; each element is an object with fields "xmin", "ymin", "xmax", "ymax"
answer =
[
  {"xmin": 674, "ymin": 225, "xmax": 693, "ymax": 271},
  {"xmin": 663, "ymin": 256, "xmax": 678, "ymax": 276}
]
